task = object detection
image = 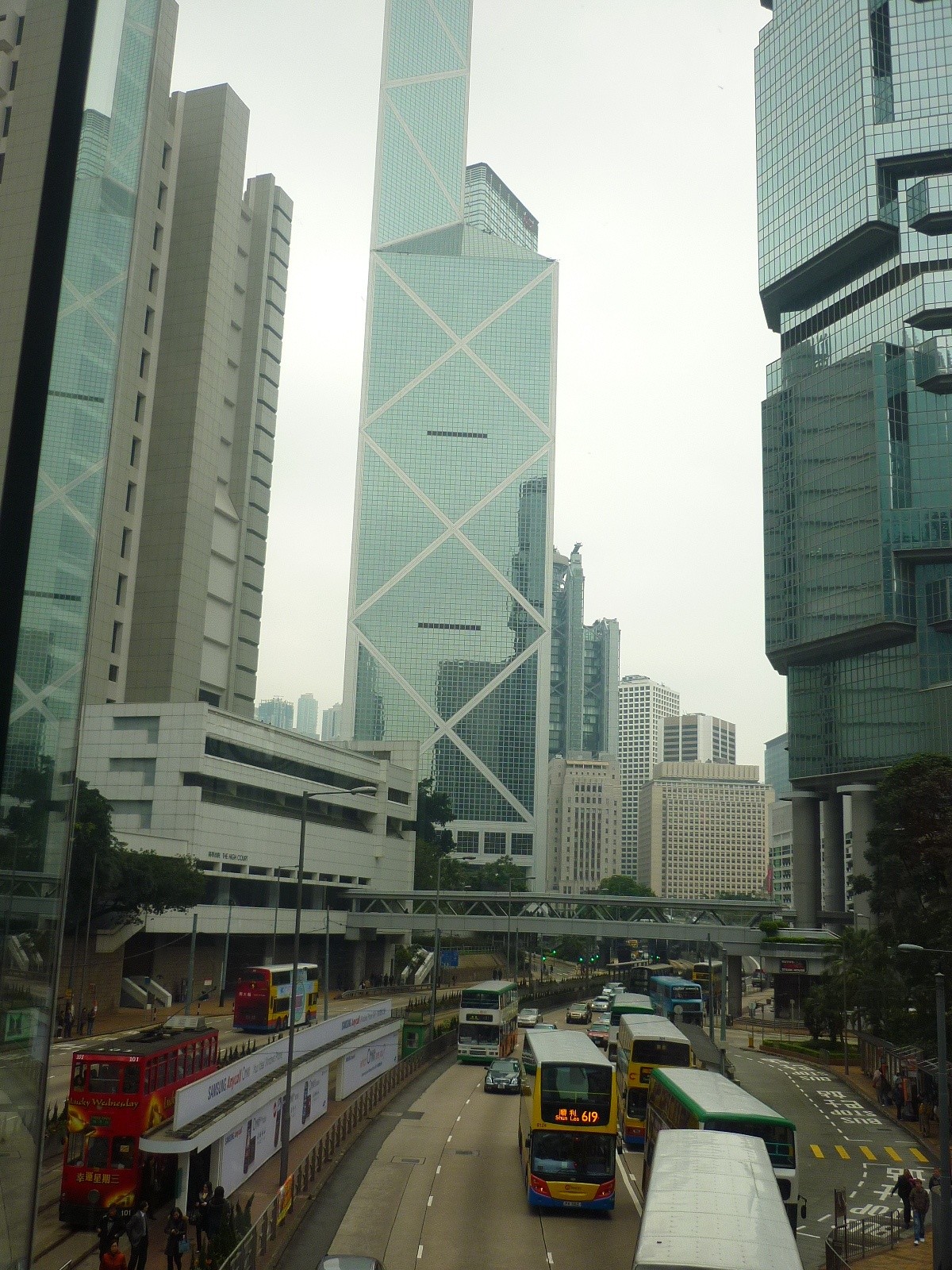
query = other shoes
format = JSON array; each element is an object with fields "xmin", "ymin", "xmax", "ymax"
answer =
[
  {"xmin": 906, "ymin": 1224, "xmax": 911, "ymax": 1229},
  {"xmin": 922, "ymin": 1133, "xmax": 927, "ymax": 1137},
  {"xmin": 920, "ymin": 1238, "xmax": 925, "ymax": 1242},
  {"xmin": 58, "ymin": 1037, "xmax": 61, "ymax": 1039},
  {"xmin": 914, "ymin": 1240, "xmax": 919, "ymax": 1245},
  {"xmin": 60, "ymin": 1037, "xmax": 63, "ymax": 1039}
]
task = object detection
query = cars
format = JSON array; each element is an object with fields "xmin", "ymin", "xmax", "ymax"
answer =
[
  {"xmin": 534, "ymin": 1024, "xmax": 557, "ymax": 1030},
  {"xmin": 598, "ymin": 1012, "xmax": 611, "ymax": 1022},
  {"xmin": 315, "ymin": 1254, "xmax": 385, "ymax": 1270},
  {"xmin": 517, "ymin": 1008, "xmax": 543, "ymax": 1028}
]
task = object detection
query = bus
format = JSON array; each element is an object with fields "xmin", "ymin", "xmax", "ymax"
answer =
[
  {"xmin": 232, "ymin": 963, "xmax": 319, "ymax": 1034},
  {"xmin": 629, "ymin": 963, "xmax": 674, "ymax": 996},
  {"xmin": 631, "ymin": 1129, "xmax": 803, "ymax": 1270},
  {"xmin": 649, "ymin": 976, "xmax": 706, "ymax": 1030},
  {"xmin": 641, "ymin": 1068, "xmax": 807, "ymax": 1241},
  {"xmin": 457, "ymin": 980, "xmax": 519, "ymax": 1063},
  {"xmin": 617, "ymin": 1013, "xmax": 696, "ymax": 1144},
  {"xmin": 692, "ymin": 961, "xmax": 723, "ymax": 1001},
  {"xmin": 608, "ymin": 993, "xmax": 655, "ymax": 1062},
  {"xmin": 58, "ymin": 985, "xmax": 219, "ymax": 1231},
  {"xmin": 518, "ymin": 1028, "xmax": 623, "ymax": 1211}
]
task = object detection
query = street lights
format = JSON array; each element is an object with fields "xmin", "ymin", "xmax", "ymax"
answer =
[
  {"xmin": 430, "ymin": 855, "xmax": 477, "ymax": 1041},
  {"xmin": 280, "ymin": 784, "xmax": 380, "ymax": 1187},
  {"xmin": 507, "ymin": 876, "xmax": 537, "ymax": 982},
  {"xmin": 855, "ymin": 913, "xmax": 871, "ymax": 933}
]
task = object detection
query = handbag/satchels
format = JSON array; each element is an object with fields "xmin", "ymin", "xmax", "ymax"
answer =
[
  {"xmin": 58, "ymin": 1026, "xmax": 63, "ymax": 1029},
  {"xmin": 363, "ymin": 983, "xmax": 365, "ymax": 986},
  {"xmin": 359, "ymin": 985, "xmax": 362, "ymax": 989},
  {"xmin": 875, "ymin": 1082, "xmax": 880, "ymax": 1089}
]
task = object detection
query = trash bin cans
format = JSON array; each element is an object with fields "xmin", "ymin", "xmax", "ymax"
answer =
[
  {"xmin": 749, "ymin": 1008, "xmax": 756, "ymax": 1016},
  {"xmin": 766, "ymin": 999, "xmax": 771, "ymax": 1004},
  {"xmin": 725, "ymin": 1014, "xmax": 734, "ymax": 1027}
]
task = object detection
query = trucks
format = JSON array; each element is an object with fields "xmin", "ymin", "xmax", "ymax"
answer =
[{"xmin": 752, "ymin": 968, "xmax": 775, "ymax": 988}]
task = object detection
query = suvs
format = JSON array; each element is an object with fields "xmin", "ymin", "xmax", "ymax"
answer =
[
  {"xmin": 587, "ymin": 1021, "xmax": 610, "ymax": 1052},
  {"xmin": 566, "ymin": 1001, "xmax": 592, "ymax": 1025},
  {"xmin": 591, "ymin": 982, "xmax": 628, "ymax": 1012},
  {"xmin": 483, "ymin": 1057, "xmax": 521, "ymax": 1095}
]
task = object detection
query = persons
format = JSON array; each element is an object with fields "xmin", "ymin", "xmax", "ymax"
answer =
[
  {"xmin": 929, "ymin": 1166, "xmax": 941, "ymax": 1192},
  {"xmin": 492, "ymin": 967, "xmax": 497, "ymax": 980},
  {"xmin": 890, "ymin": 1168, "xmax": 916, "ymax": 1229},
  {"xmin": 909, "ymin": 1179, "xmax": 930, "ymax": 1245},
  {"xmin": 872, "ymin": 1068, "xmax": 931, "ymax": 1138},
  {"xmin": 57, "ymin": 1006, "xmax": 96, "ymax": 1038},
  {"xmin": 359, "ymin": 973, "xmax": 393, "ymax": 993},
  {"xmin": 96, "ymin": 1182, "xmax": 232, "ymax": 1270},
  {"xmin": 497, "ymin": 969, "xmax": 502, "ymax": 981}
]
